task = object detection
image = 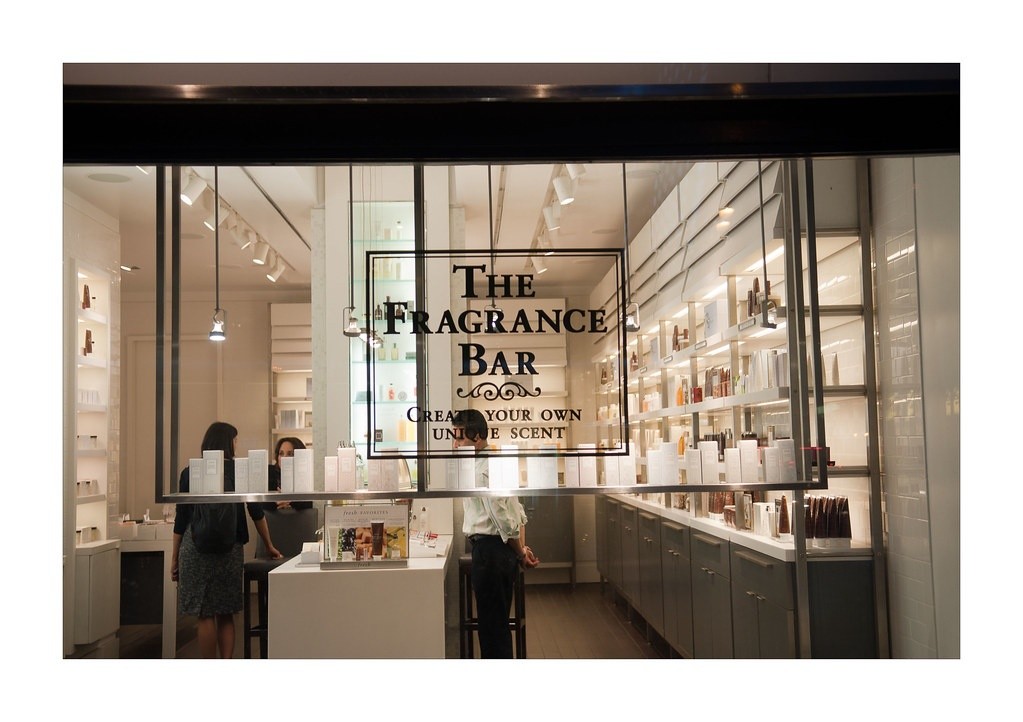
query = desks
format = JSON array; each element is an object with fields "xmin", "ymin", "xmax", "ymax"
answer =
[
  {"xmin": 268, "ymin": 535, "xmax": 454, "ymax": 660},
  {"xmin": 121, "ymin": 512, "xmax": 182, "ymax": 659}
]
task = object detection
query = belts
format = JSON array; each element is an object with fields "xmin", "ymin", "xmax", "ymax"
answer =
[{"xmin": 468, "ymin": 534, "xmax": 487, "ymax": 544}]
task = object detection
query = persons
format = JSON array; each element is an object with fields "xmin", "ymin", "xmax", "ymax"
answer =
[
  {"xmin": 255, "ymin": 437, "xmax": 313, "ymax": 636},
  {"xmin": 452, "ymin": 409, "xmax": 539, "ymax": 659},
  {"xmin": 170, "ymin": 422, "xmax": 284, "ymax": 659}
]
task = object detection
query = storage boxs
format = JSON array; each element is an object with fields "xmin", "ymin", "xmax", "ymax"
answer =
[{"xmin": 187, "ymin": 439, "xmax": 798, "ymax": 492}]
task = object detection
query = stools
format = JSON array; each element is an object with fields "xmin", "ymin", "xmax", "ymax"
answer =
[
  {"xmin": 458, "ymin": 552, "xmax": 528, "ymax": 659},
  {"xmin": 244, "ymin": 558, "xmax": 286, "ymax": 659}
]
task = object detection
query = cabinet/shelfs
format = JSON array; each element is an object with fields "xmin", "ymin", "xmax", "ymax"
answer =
[
  {"xmin": 264, "ymin": 300, "xmax": 579, "ymax": 588},
  {"xmin": 63, "ymin": 187, "xmax": 121, "ymax": 655},
  {"xmin": 580, "ymin": 157, "xmax": 886, "ymax": 658}
]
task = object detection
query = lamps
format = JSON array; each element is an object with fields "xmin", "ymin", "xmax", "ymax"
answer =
[
  {"xmin": 619, "ymin": 163, "xmax": 640, "ymax": 332},
  {"xmin": 340, "ymin": 164, "xmax": 363, "ymax": 337},
  {"xmin": 180, "ymin": 165, "xmax": 286, "ymax": 340},
  {"xmin": 481, "ymin": 164, "xmax": 503, "ymax": 330},
  {"xmin": 530, "ymin": 164, "xmax": 586, "ymax": 275}
]
